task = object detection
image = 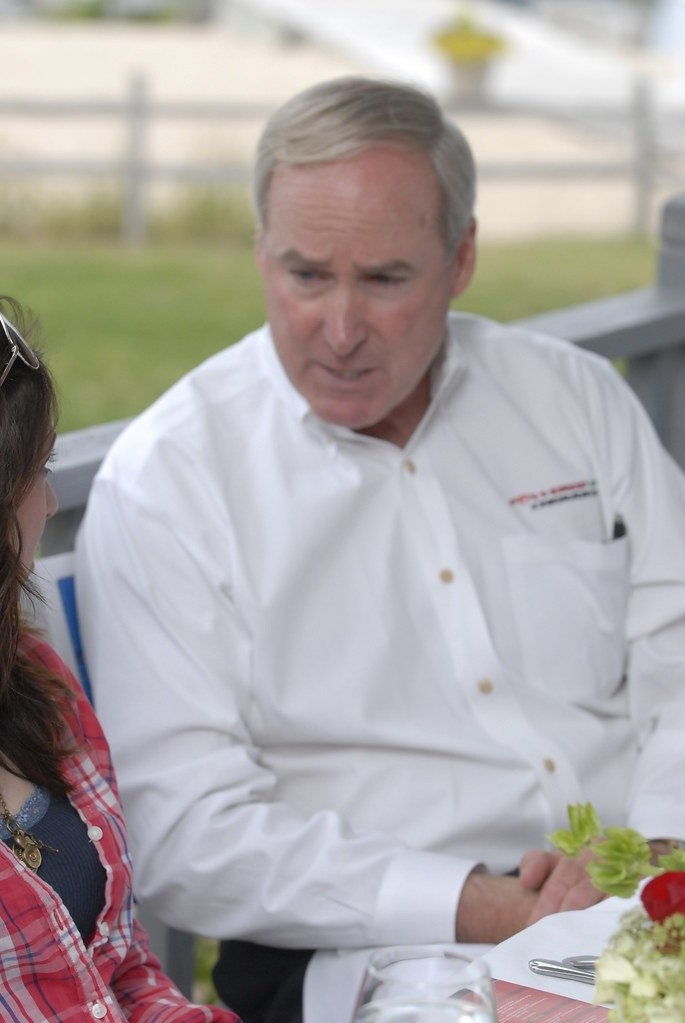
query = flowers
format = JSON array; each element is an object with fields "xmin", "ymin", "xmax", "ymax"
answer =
[{"xmin": 548, "ymin": 801, "xmax": 685, "ymax": 1023}]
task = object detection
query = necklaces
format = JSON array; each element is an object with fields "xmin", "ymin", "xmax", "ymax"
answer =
[{"xmin": 0, "ymin": 794, "xmax": 43, "ymax": 876}]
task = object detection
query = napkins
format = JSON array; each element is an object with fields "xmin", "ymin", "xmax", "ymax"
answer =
[{"xmin": 467, "ymin": 875, "xmax": 657, "ymax": 1010}]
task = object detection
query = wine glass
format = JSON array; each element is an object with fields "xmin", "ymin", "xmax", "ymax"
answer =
[{"xmin": 353, "ymin": 945, "xmax": 497, "ymax": 1023}]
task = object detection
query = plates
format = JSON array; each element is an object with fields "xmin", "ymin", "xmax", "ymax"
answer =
[{"xmin": 480, "ymin": 875, "xmax": 652, "ymax": 1009}]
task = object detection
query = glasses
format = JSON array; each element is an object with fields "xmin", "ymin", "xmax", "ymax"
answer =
[{"xmin": 0, "ymin": 311, "xmax": 40, "ymax": 386}]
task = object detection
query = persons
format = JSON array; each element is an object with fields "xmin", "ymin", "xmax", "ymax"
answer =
[
  {"xmin": 73, "ymin": 76, "xmax": 685, "ymax": 1023},
  {"xmin": 0, "ymin": 314, "xmax": 241, "ymax": 1022}
]
